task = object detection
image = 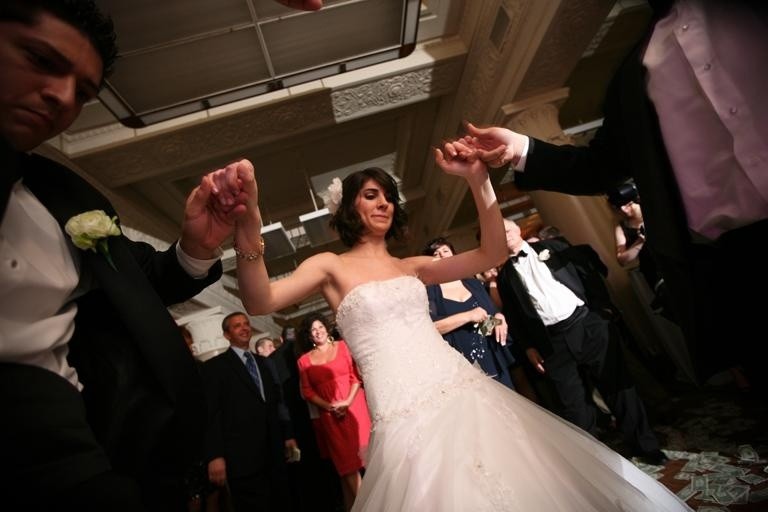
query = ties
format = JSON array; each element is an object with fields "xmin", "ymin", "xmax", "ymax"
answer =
[{"xmin": 244, "ymin": 352, "xmax": 260, "ymax": 392}]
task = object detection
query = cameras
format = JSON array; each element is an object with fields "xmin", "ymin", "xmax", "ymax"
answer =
[{"xmin": 606, "ymin": 177, "xmax": 638, "ymax": 209}]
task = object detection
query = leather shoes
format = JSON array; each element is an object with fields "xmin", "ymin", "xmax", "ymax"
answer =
[
  {"xmin": 639, "ymin": 448, "xmax": 668, "ymax": 465},
  {"xmin": 656, "ymin": 404, "xmax": 676, "ymax": 426}
]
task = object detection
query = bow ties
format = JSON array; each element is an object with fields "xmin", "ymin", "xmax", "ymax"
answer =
[{"xmin": 511, "ymin": 250, "xmax": 527, "ymax": 263}]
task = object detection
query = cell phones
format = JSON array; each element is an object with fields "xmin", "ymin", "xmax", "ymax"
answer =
[{"xmin": 291, "ymin": 448, "xmax": 301, "ymax": 462}]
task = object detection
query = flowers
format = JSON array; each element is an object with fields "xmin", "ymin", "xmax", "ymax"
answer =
[
  {"xmin": 63, "ymin": 208, "xmax": 123, "ymax": 274},
  {"xmin": 317, "ymin": 178, "xmax": 343, "ymax": 217}
]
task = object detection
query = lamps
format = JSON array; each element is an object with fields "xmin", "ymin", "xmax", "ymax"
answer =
[{"xmin": 261, "ymin": 176, "xmax": 338, "ymax": 262}]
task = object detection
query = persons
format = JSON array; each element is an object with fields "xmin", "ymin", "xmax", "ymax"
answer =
[
  {"xmin": 208, "ymin": 137, "xmax": 696, "ymax": 511},
  {"xmin": 457, "ymin": 1, "xmax": 768, "ymax": 437},
  {"xmin": 423, "ymin": 178, "xmax": 712, "ymax": 466},
  {"xmin": 177, "ymin": 312, "xmax": 372, "ymax": 511},
  {"xmin": 0, "ymin": 1, "xmax": 250, "ymax": 511}
]
